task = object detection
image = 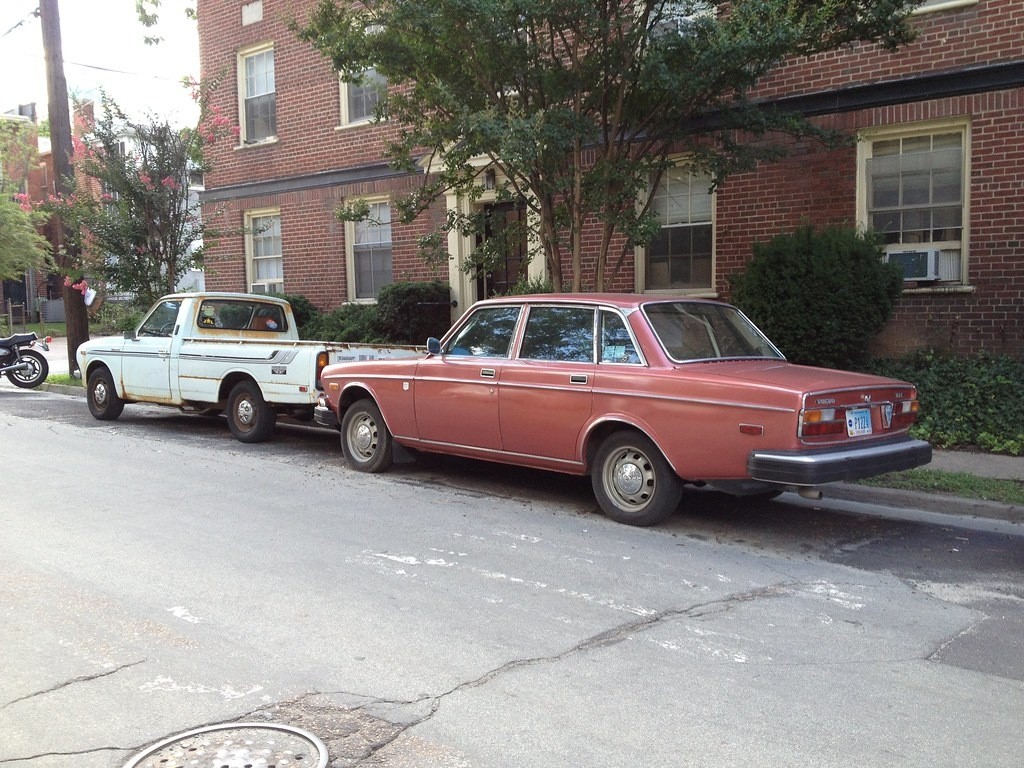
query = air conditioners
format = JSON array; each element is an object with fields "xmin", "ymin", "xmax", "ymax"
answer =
[{"xmin": 886, "ymin": 249, "xmax": 940, "ymax": 283}]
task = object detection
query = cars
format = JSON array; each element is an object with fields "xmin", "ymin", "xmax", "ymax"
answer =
[{"xmin": 313, "ymin": 290, "xmax": 933, "ymax": 529}]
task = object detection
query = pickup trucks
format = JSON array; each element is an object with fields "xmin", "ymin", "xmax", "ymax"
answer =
[{"xmin": 75, "ymin": 291, "xmax": 439, "ymax": 444}]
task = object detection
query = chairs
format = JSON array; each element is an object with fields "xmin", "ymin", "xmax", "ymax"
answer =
[{"xmin": 249, "ymin": 316, "xmax": 274, "ymax": 331}]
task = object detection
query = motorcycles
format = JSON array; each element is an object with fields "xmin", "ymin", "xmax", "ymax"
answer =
[{"xmin": 0, "ymin": 332, "xmax": 49, "ymax": 389}]
